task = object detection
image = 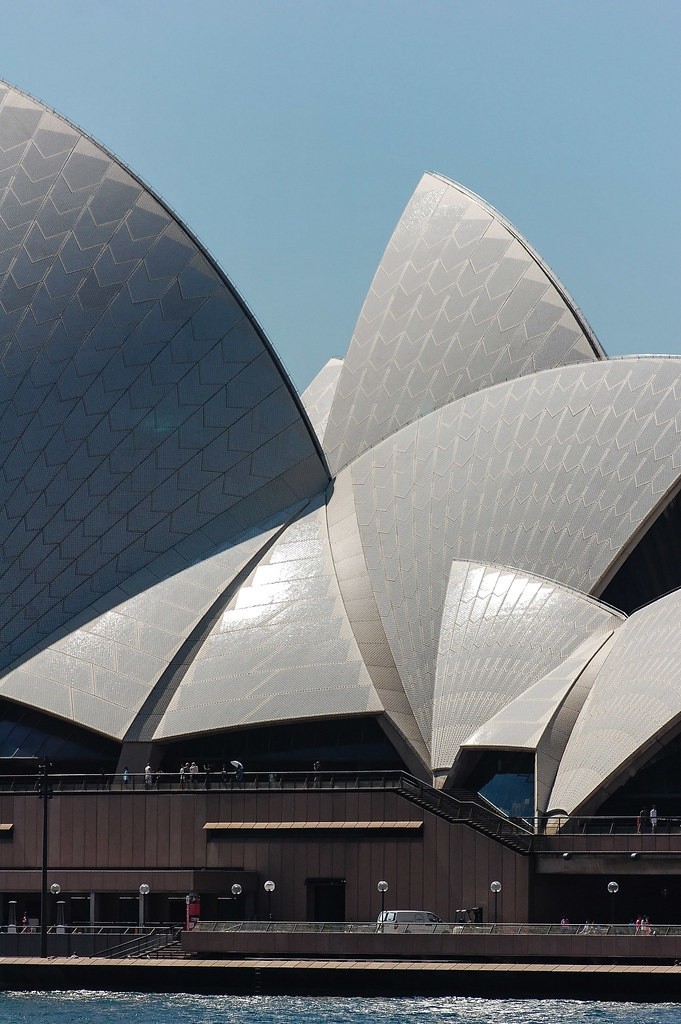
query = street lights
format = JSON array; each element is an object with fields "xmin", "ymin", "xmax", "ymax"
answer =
[
  {"xmin": 265, "ymin": 881, "xmax": 275, "ymax": 921},
  {"xmin": 232, "ymin": 885, "xmax": 243, "ymax": 919},
  {"xmin": 36, "ymin": 755, "xmax": 55, "ymax": 957},
  {"xmin": 377, "ymin": 881, "xmax": 389, "ymax": 932},
  {"xmin": 608, "ymin": 881, "xmax": 619, "ymax": 930},
  {"xmin": 139, "ymin": 884, "xmax": 149, "ymax": 934},
  {"xmin": 50, "ymin": 883, "xmax": 60, "ymax": 933},
  {"xmin": 491, "ymin": 881, "xmax": 501, "ymax": 922}
]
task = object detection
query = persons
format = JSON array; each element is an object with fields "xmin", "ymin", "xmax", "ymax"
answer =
[
  {"xmin": 190, "ymin": 762, "xmax": 198, "ymax": 788},
  {"xmin": 560, "ymin": 914, "xmax": 570, "ymax": 933},
  {"xmin": 179, "ymin": 762, "xmax": 190, "ymax": 789},
  {"xmin": 650, "ymin": 804, "xmax": 658, "ymax": 834},
  {"xmin": 144, "ymin": 762, "xmax": 153, "ymax": 790},
  {"xmin": 202, "ymin": 764, "xmax": 211, "ymax": 789},
  {"xmin": 635, "ymin": 914, "xmax": 652, "ymax": 936},
  {"xmin": 22, "ymin": 911, "xmax": 32, "ymax": 933},
  {"xmin": 236, "ymin": 764, "xmax": 243, "ymax": 789},
  {"xmin": 312, "ymin": 761, "xmax": 320, "ymax": 788},
  {"xmin": 122, "ymin": 765, "xmax": 131, "ymax": 787},
  {"xmin": 639, "ymin": 806, "xmax": 649, "ymax": 834}
]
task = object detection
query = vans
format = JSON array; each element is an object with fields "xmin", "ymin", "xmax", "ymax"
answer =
[{"xmin": 377, "ymin": 910, "xmax": 452, "ymax": 934}]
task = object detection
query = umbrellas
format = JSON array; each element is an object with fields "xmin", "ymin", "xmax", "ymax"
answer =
[{"xmin": 230, "ymin": 761, "xmax": 243, "ymax": 769}]
